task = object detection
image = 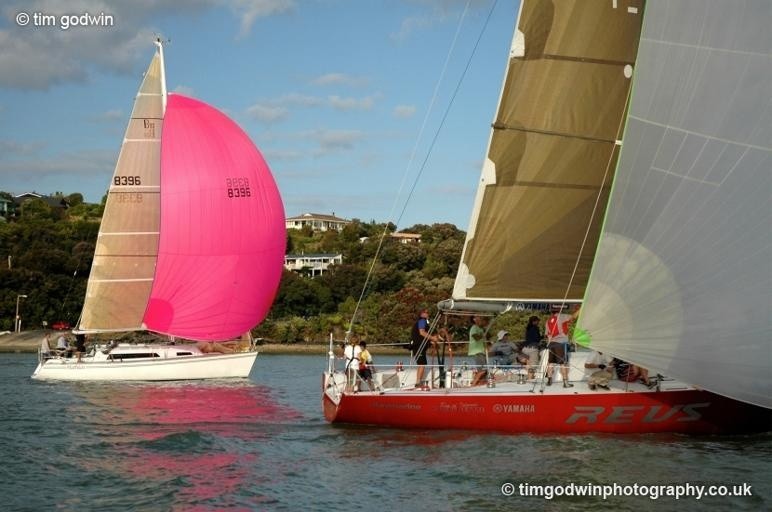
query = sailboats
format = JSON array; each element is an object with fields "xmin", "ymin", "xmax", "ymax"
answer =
[
  {"xmin": 29, "ymin": 29, "xmax": 297, "ymax": 384},
  {"xmin": 321, "ymin": 1, "xmax": 772, "ymax": 436}
]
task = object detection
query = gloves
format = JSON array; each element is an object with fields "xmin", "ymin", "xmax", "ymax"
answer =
[{"xmin": 598, "ymin": 364, "xmax": 606, "ymax": 370}]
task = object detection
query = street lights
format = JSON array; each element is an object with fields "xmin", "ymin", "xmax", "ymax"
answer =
[{"xmin": 15, "ymin": 294, "xmax": 28, "ymax": 332}]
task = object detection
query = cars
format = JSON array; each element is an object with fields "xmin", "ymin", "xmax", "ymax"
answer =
[{"xmin": 50, "ymin": 319, "xmax": 71, "ymax": 331}]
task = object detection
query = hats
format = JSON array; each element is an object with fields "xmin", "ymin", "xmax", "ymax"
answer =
[
  {"xmin": 528, "ymin": 315, "xmax": 540, "ymax": 324},
  {"xmin": 496, "ymin": 329, "xmax": 511, "ymax": 341}
]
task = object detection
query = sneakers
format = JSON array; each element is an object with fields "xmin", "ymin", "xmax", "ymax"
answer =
[
  {"xmin": 563, "ymin": 383, "xmax": 575, "ymax": 388},
  {"xmin": 589, "ymin": 384, "xmax": 596, "ymax": 390},
  {"xmin": 548, "ymin": 376, "xmax": 553, "ymax": 385},
  {"xmin": 647, "ymin": 380, "xmax": 657, "ymax": 390},
  {"xmin": 597, "ymin": 383, "xmax": 610, "ymax": 391}
]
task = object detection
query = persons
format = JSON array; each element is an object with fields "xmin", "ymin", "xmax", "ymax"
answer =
[
  {"xmin": 466, "ymin": 313, "xmax": 490, "ymax": 387},
  {"xmin": 583, "ymin": 350, "xmax": 614, "ymax": 391},
  {"xmin": 40, "ymin": 331, "xmax": 91, "ymax": 359},
  {"xmin": 341, "ymin": 334, "xmax": 360, "ymax": 393},
  {"xmin": 610, "ymin": 357, "xmax": 656, "ymax": 390},
  {"xmin": 355, "ymin": 340, "xmax": 376, "ymax": 394},
  {"xmin": 412, "ymin": 306, "xmax": 439, "ymax": 390},
  {"xmin": 524, "ymin": 315, "xmax": 546, "ymax": 384},
  {"xmin": 543, "ymin": 304, "xmax": 580, "ymax": 388},
  {"xmin": 487, "ymin": 329, "xmax": 530, "ymax": 371}
]
